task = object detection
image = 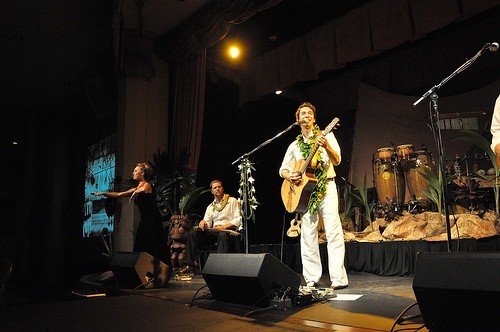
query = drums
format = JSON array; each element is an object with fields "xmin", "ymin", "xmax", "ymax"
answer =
[
  {"xmin": 398, "ymin": 145, "xmax": 415, "ymax": 162},
  {"xmin": 377, "ymin": 147, "xmax": 394, "ymax": 162},
  {"xmin": 371, "ymin": 159, "xmax": 406, "ymax": 212},
  {"xmin": 404, "ymin": 151, "xmax": 437, "ymax": 205}
]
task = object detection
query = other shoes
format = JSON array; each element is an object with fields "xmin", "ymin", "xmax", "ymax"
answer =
[
  {"xmin": 180, "ymin": 266, "xmax": 195, "ymax": 273},
  {"xmin": 330, "ymin": 285, "xmax": 348, "ymax": 290},
  {"xmin": 307, "ymin": 282, "xmax": 318, "ymax": 287}
]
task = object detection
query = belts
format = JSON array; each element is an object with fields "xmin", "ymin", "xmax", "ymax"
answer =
[{"xmin": 326, "ymin": 176, "xmax": 336, "ymax": 181}]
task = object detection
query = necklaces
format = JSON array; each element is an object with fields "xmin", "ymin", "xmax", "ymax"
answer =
[
  {"xmin": 211, "ymin": 194, "xmax": 229, "ymax": 211},
  {"xmin": 296, "ymin": 125, "xmax": 328, "ymax": 215}
]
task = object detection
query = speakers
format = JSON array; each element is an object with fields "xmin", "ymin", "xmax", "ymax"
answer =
[
  {"xmin": 110, "ymin": 251, "xmax": 170, "ymax": 288},
  {"xmin": 412, "ymin": 252, "xmax": 500, "ymax": 332},
  {"xmin": 202, "ymin": 253, "xmax": 302, "ymax": 308}
]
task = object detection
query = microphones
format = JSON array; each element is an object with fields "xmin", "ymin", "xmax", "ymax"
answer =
[
  {"xmin": 293, "ymin": 118, "xmax": 309, "ymax": 125},
  {"xmin": 487, "ymin": 42, "xmax": 499, "ymax": 52}
]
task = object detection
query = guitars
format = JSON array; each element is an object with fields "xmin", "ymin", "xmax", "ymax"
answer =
[
  {"xmin": 280, "ymin": 118, "xmax": 339, "ymax": 213},
  {"xmin": 286, "ymin": 213, "xmax": 301, "ymax": 237},
  {"xmin": 191, "ymin": 225, "xmax": 241, "ymax": 237}
]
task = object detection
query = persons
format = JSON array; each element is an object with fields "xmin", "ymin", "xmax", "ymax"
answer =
[
  {"xmin": 489, "ymin": 93, "xmax": 500, "ymax": 171},
  {"xmin": 90, "ymin": 161, "xmax": 171, "ymax": 265},
  {"xmin": 177, "ymin": 180, "xmax": 245, "ymax": 275},
  {"xmin": 275, "ymin": 102, "xmax": 352, "ymax": 291}
]
